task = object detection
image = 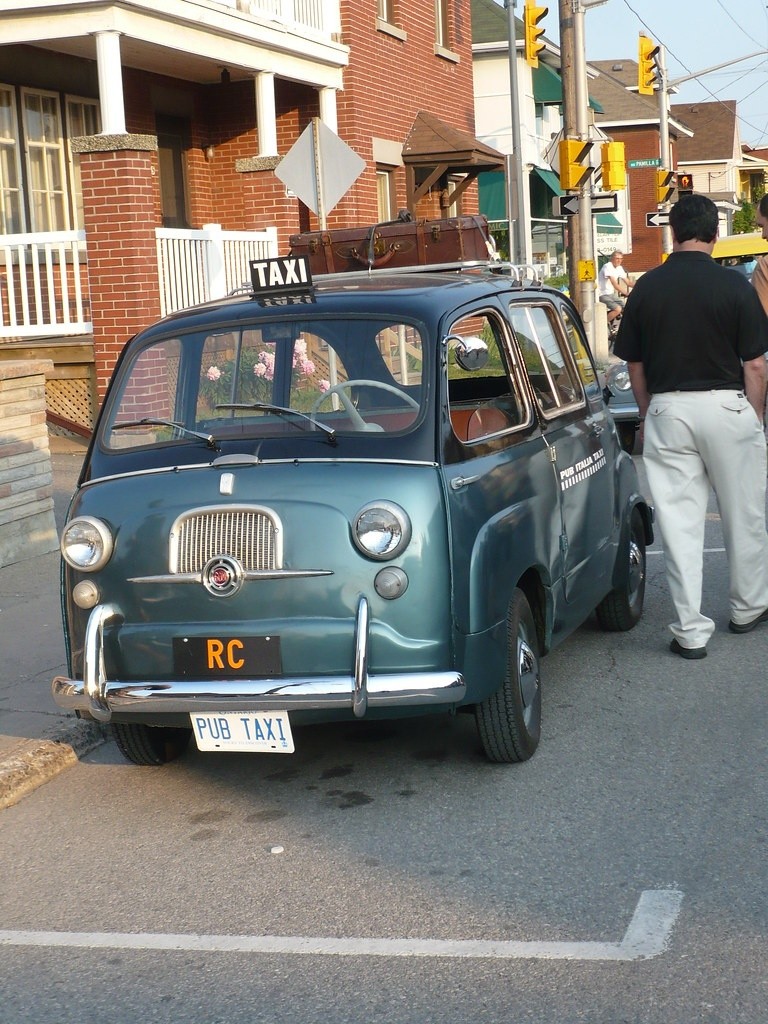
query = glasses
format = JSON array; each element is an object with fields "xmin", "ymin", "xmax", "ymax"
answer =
[{"xmin": 615, "ymin": 257, "xmax": 624, "ymax": 260}]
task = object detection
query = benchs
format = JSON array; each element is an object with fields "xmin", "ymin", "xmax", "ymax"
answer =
[{"xmin": 211, "ymin": 402, "xmax": 516, "ymax": 442}]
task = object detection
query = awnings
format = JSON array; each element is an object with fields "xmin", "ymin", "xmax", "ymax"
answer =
[
  {"xmin": 477, "ymin": 163, "xmax": 624, "ymax": 234},
  {"xmin": 532, "ymin": 59, "xmax": 606, "ymax": 115}
]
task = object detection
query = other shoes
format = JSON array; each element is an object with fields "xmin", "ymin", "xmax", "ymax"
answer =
[
  {"xmin": 670, "ymin": 635, "xmax": 708, "ymax": 660},
  {"xmin": 607, "ymin": 318, "xmax": 614, "ymax": 337},
  {"xmin": 728, "ymin": 610, "xmax": 767, "ymax": 634}
]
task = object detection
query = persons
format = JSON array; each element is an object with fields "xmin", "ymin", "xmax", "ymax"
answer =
[
  {"xmin": 598, "ymin": 249, "xmax": 636, "ymax": 325},
  {"xmin": 751, "ymin": 193, "xmax": 768, "ymax": 320},
  {"xmin": 612, "ymin": 194, "xmax": 768, "ymax": 659}
]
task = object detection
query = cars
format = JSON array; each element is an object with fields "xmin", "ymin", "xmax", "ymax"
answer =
[
  {"xmin": 600, "ymin": 232, "xmax": 768, "ymax": 453},
  {"xmin": 52, "ymin": 253, "xmax": 655, "ymax": 764}
]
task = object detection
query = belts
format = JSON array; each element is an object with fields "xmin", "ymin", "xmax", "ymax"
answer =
[{"xmin": 710, "ymin": 381, "xmax": 745, "ymax": 393}]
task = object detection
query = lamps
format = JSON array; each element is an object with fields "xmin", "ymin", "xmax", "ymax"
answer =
[
  {"xmin": 202, "ymin": 141, "xmax": 215, "ymax": 163},
  {"xmin": 221, "ymin": 68, "xmax": 231, "ymax": 87},
  {"xmin": 442, "ymin": 189, "xmax": 450, "ymax": 207}
]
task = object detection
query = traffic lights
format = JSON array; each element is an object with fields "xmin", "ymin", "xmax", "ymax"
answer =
[
  {"xmin": 655, "ymin": 169, "xmax": 675, "ymax": 204},
  {"xmin": 677, "ymin": 173, "xmax": 694, "ymax": 199},
  {"xmin": 638, "ymin": 36, "xmax": 660, "ymax": 95},
  {"xmin": 559, "ymin": 139, "xmax": 596, "ymax": 192},
  {"xmin": 525, "ymin": 0, "xmax": 548, "ymax": 68}
]
